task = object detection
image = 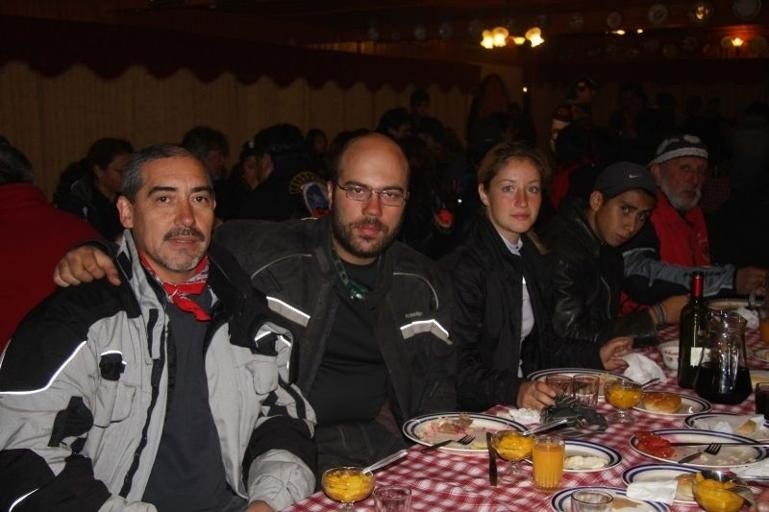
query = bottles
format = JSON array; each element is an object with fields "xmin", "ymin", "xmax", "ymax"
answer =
[{"xmin": 677, "ymin": 271, "xmax": 710, "ymax": 389}]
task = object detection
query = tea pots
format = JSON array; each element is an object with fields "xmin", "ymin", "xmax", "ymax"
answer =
[{"xmin": 695, "ymin": 309, "xmax": 752, "ymax": 405}]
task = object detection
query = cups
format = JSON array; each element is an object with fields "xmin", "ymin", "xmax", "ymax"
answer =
[
  {"xmin": 573, "ymin": 376, "xmax": 599, "ymax": 409},
  {"xmin": 571, "ymin": 489, "xmax": 613, "ymax": 512},
  {"xmin": 371, "ymin": 485, "xmax": 413, "ymax": 512},
  {"xmin": 534, "ymin": 433, "xmax": 566, "ymax": 490},
  {"xmin": 546, "ymin": 374, "xmax": 573, "ymax": 398},
  {"xmin": 754, "ymin": 382, "xmax": 769, "ymax": 420}
]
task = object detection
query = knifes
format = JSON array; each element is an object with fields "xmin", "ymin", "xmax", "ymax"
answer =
[
  {"xmin": 485, "ymin": 432, "xmax": 497, "ymax": 487},
  {"xmin": 668, "ymin": 441, "xmax": 769, "ymax": 449}
]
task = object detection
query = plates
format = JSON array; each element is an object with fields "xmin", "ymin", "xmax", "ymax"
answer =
[
  {"xmin": 629, "ymin": 428, "xmax": 769, "ymax": 467},
  {"xmin": 526, "ymin": 439, "xmax": 623, "ymax": 473},
  {"xmin": 402, "ymin": 413, "xmax": 531, "ymax": 452},
  {"xmin": 633, "ymin": 392, "xmax": 712, "ymax": 416},
  {"xmin": 708, "ymin": 299, "xmax": 760, "ymax": 310},
  {"xmin": 684, "ymin": 411, "xmax": 769, "ymax": 442},
  {"xmin": 528, "ymin": 366, "xmax": 634, "ymax": 399},
  {"xmin": 621, "ymin": 464, "xmax": 720, "ymax": 504},
  {"xmin": 550, "ymin": 486, "xmax": 673, "ymax": 512}
]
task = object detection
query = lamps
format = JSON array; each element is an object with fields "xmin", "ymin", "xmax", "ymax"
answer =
[{"xmin": 479, "ymin": 0, "xmax": 546, "ymax": 50}]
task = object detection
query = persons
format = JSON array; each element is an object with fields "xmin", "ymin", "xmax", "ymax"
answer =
[
  {"xmin": 2, "ymin": 68, "xmax": 768, "ymax": 241},
  {"xmin": 0, "ymin": 145, "xmax": 322, "ymax": 510},
  {"xmin": 533, "ymin": 165, "xmax": 701, "ymax": 348},
  {"xmin": 53, "ymin": 126, "xmax": 455, "ymax": 493},
  {"xmin": 0, "ymin": 136, "xmax": 104, "ymax": 358},
  {"xmin": 436, "ymin": 139, "xmax": 634, "ymax": 413},
  {"xmin": 623, "ymin": 126, "xmax": 769, "ymax": 305}
]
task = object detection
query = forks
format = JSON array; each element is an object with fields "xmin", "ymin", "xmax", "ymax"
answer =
[
  {"xmin": 679, "ymin": 439, "xmax": 722, "ymax": 462},
  {"xmin": 420, "ymin": 435, "xmax": 477, "ymax": 453}
]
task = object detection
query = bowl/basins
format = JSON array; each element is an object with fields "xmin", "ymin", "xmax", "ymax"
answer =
[{"xmin": 657, "ymin": 340, "xmax": 679, "ymax": 370}]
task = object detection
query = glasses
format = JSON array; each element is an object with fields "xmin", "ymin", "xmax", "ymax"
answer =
[
  {"xmin": 332, "ymin": 181, "xmax": 411, "ymax": 206},
  {"xmin": 547, "ymin": 410, "xmax": 607, "ymax": 437}
]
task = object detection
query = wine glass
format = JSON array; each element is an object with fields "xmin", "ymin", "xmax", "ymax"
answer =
[
  {"xmin": 490, "ymin": 427, "xmax": 535, "ymax": 482},
  {"xmin": 605, "ymin": 379, "xmax": 643, "ymax": 422},
  {"xmin": 321, "ymin": 467, "xmax": 374, "ymax": 512}
]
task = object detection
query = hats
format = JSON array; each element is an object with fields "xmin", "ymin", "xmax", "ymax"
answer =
[
  {"xmin": 646, "ymin": 129, "xmax": 710, "ymax": 170},
  {"xmin": 594, "ymin": 161, "xmax": 658, "ymax": 201}
]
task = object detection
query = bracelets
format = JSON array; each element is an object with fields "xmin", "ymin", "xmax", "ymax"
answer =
[{"xmin": 647, "ymin": 296, "xmax": 669, "ymax": 327}]
